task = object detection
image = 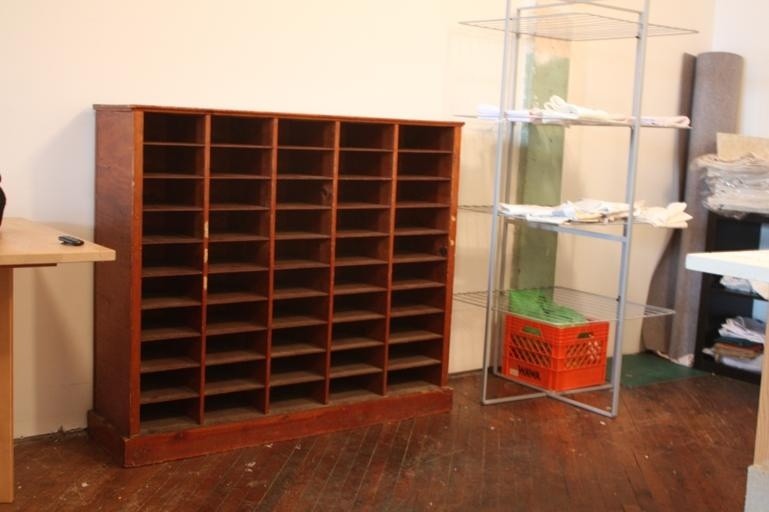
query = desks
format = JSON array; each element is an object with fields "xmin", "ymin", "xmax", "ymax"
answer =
[
  {"xmin": 684, "ymin": 248, "xmax": 769, "ymax": 512},
  {"xmin": 1, "ymin": 219, "xmax": 116, "ymax": 502}
]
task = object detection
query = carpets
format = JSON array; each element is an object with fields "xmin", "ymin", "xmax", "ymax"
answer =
[{"xmin": 604, "ymin": 352, "xmax": 715, "ymax": 391}]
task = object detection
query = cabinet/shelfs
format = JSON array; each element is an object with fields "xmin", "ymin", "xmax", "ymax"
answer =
[
  {"xmin": 455, "ymin": 0, "xmax": 702, "ymax": 421},
  {"xmin": 86, "ymin": 103, "xmax": 465, "ymax": 469}
]
